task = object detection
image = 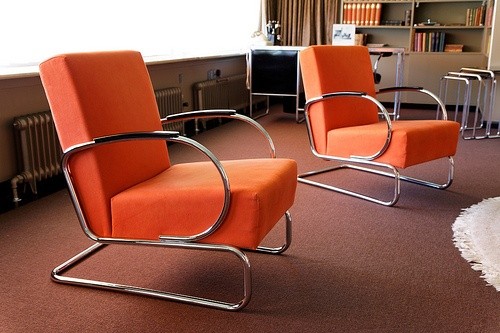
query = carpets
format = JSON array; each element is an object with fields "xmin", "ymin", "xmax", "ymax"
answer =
[{"xmin": 450, "ymin": 196, "xmax": 500, "ymax": 292}]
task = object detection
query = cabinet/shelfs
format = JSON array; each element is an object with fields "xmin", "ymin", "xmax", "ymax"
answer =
[{"xmin": 341, "ymin": 0, "xmax": 500, "ymax": 126}]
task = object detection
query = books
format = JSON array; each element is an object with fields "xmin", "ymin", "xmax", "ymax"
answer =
[
  {"xmin": 343, "ymin": 3, "xmax": 411, "ymax": 26},
  {"xmin": 466, "ymin": 0, "xmax": 495, "ymax": 27},
  {"xmin": 410, "ymin": 28, "xmax": 464, "ymax": 53}
]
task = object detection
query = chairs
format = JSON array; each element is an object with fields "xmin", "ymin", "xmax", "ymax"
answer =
[
  {"xmin": 245, "ymin": 47, "xmax": 305, "ymax": 124},
  {"xmin": 38, "ymin": 50, "xmax": 299, "ymax": 311},
  {"xmin": 298, "ymin": 45, "xmax": 461, "ymax": 207}
]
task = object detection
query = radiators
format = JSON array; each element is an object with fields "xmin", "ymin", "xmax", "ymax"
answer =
[
  {"xmin": 194, "ymin": 73, "xmax": 249, "ymax": 121},
  {"xmin": 154, "ymin": 88, "xmax": 185, "ymax": 135},
  {"xmin": 11, "ymin": 113, "xmax": 62, "ymax": 203}
]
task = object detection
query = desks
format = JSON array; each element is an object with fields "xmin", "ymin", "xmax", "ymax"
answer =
[{"xmin": 369, "ymin": 48, "xmax": 406, "ymax": 118}]
